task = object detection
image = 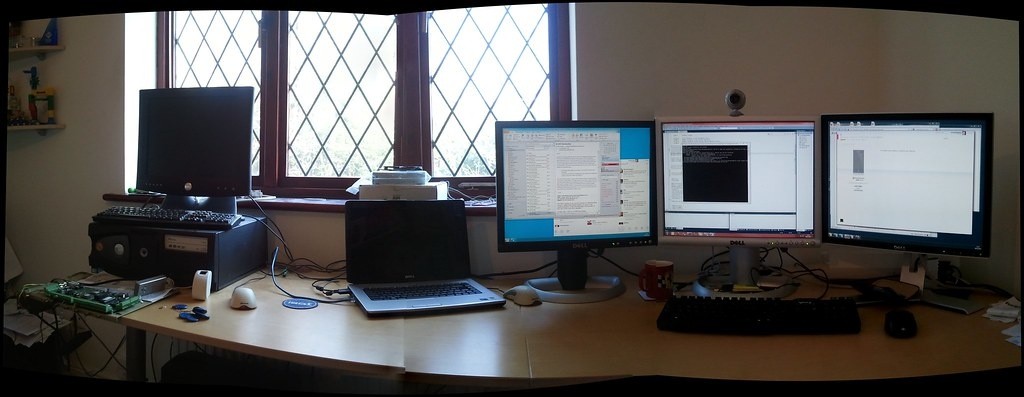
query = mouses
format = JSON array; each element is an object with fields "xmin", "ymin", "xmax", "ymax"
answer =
[
  {"xmin": 503, "ymin": 286, "xmax": 542, "ymax": 306},
  {"xmin": 230, "ymin": 287, "xmax": 257, "ymax": 310},
  {"xmin": 884, "ymin": 310, "xmax": 919, "ymax": 339}
]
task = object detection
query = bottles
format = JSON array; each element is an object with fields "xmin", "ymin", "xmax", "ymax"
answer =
[{"xmin": 8, "ymin": 85, "xmax": 17, "ymax": 115}]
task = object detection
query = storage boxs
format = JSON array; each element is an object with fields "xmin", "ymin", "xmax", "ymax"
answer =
[
  {"xmin": 372, "ymin": 171, "xmax": 427, "ymax": 184},
  {"xmin": 359, "ymin": 180, "xmax": 447, "ymax": 200}
]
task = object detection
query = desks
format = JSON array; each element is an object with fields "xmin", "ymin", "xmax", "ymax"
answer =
[
  {"xmin": 26, "ymin": 262, "xmax": 406, "ymax": 397},
  {"xmin": 405, "ymin": 269, "xmax": 1024, "ymax": 397}
]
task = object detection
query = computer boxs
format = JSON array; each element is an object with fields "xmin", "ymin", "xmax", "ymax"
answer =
[{"xmin": 86, "ymin": 214, "xmax": 269, "ymax": 291}]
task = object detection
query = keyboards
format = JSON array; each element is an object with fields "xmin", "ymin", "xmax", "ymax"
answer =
[
  {"xmin": 92, "ymin": 206, "xmax": 243, "ymax": 231},
  {"xmin": 655, "ymin": 297, "xmax": 861, "ymax": 336}
]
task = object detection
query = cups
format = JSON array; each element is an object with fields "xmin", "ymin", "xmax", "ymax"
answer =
[{"xmin": 639, "ymin": 260, "xmax": 673, "ymax": 300}]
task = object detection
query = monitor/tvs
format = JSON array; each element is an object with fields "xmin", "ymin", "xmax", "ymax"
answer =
[
  {"xmin": 494, "ymin": 121, "xmax": 657, "ymax": 304},
  {"xmin": 820, "ymin": 112, "xmax": 994, "ymax": 314},
  {"xmin": 656, "ymin": 117, "xmax": 823, "ymax": 298},
  {"xmin": 137, "ymin": 87, "xmax": 254, "ymax": 213}
]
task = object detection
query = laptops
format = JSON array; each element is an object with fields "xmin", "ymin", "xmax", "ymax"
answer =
[{"xmin": 344, "ymin": 200, "xmax": 506, "ymax": 320}]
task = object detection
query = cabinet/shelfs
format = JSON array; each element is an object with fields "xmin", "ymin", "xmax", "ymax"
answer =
[{"xmin": 6, "ymin": 45, "xmax": 65, "ymax": 135}]
